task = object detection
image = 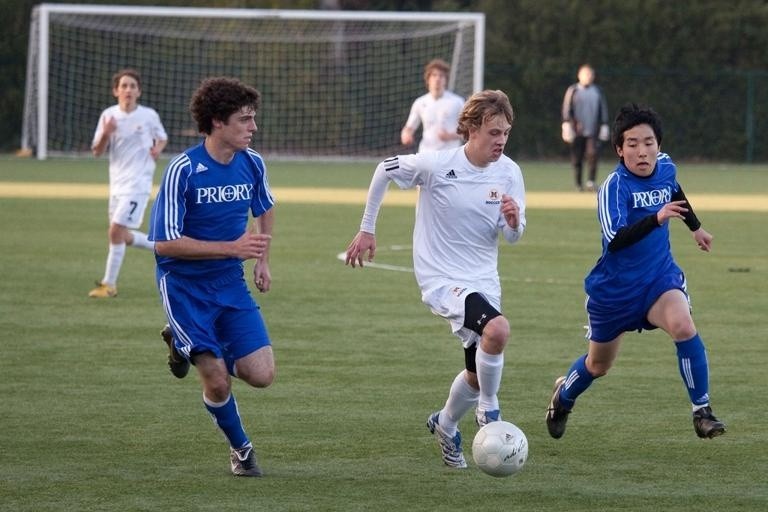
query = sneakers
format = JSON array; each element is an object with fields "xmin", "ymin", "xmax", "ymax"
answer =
[
  {"xmin": 546, "ymin": 375, "xmax": 575, "ymax": 439},
  {"xmin": 427, "ymin": 411, "xmax": 467, "ymax": 469},
  {"xmin": 89, "ymin": 284, "xmax": 118, "ymax": 298},
  {"xmin": 475, "ymin": 406, "xmax": 502, "ymax": 429},
  {"xmin": 230, "ymin": 444, "xmax": 262, "ymax": 478},
  {"xmin": 160, "ymin": 323, "xmax": 191, "ymax": 379},
  {"xmin": 692, "ymin": 406, "xmax": 724, "ymax": 439}
]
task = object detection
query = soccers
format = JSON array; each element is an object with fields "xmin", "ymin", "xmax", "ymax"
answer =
[{"xmin": 473, "ymin": 421, "xmax": 528, "ymax": 477}]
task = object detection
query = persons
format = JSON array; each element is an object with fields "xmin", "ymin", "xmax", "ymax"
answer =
[
  {"xmin": 88, "ymin": 70, "xmax": 169, "ymax": 299},
  {"xmin": 148, "ymin": 76, "xmax": 277, "ymax": 479},
  {"xmin": 545, "ymin": 100, "xmax": 727, "ymax": 440},
  {"xmin": 561, "ymin": 63, "xmax": 611, "ymax": 194},
  {"xmin": 344, "ymin": 88, "xmax": 527, "ymax": 469},
  {"xmin": 400, "ymin": 57, "xmax": 466, "ymax": 155}
]
